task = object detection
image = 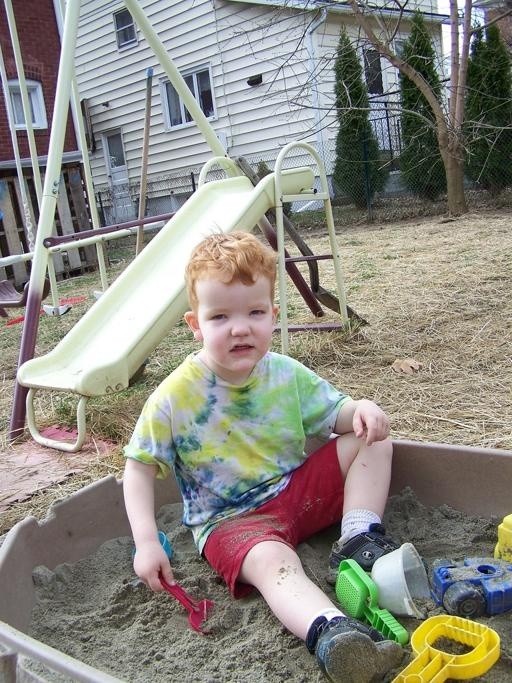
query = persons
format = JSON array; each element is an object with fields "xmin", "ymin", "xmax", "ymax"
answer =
[{"xmin": 120, "ymin": 230, "xmax": 406, "ymax": 683}]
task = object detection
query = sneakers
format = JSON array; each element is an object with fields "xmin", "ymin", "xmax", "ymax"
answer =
[
  {"xmin": 326, "ymin": 524, "xmax": 428, "ymax": 586},
  {"xmin": 306, "ymin": 616, "xmax": 404, "ymax": 683}
]
